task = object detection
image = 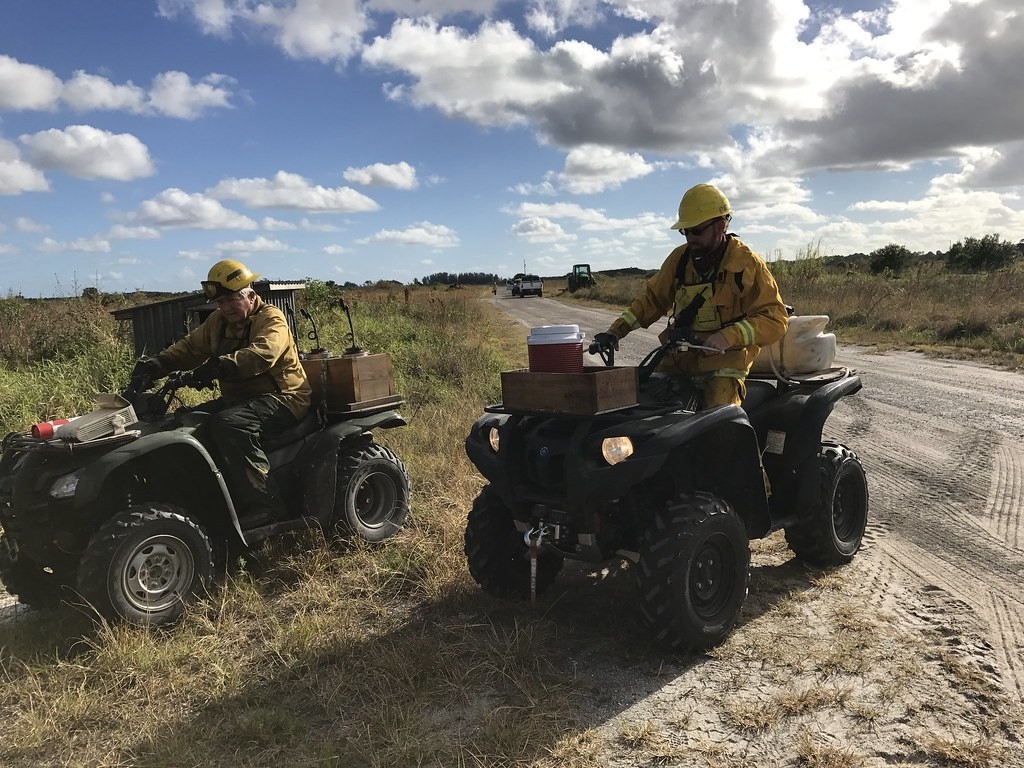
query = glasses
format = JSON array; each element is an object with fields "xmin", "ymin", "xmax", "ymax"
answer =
[{"xmin": 681, "ymin": 221, "xmax": 722, "ymax": 237}]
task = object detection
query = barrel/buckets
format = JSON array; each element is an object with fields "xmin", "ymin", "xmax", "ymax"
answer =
[{"xmin": 525, "ymin": 324, "xmax": 584, "ymax": 374}]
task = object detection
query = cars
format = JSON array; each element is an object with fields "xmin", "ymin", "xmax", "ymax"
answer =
[{"xmin": 506, "ymin": 278, "xmax": 522, "ymax": 291}]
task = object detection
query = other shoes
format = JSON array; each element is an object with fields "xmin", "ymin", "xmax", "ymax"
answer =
[{"xmin": 239, "ymin": 493, "xmax": 289, "ymax": 530}]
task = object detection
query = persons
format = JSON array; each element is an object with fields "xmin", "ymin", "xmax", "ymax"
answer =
[
  {"xmin": 492, "ymin": 281, "xmax": 499, "ymax": 296},
  {"xmin": 590, "ymin": 184, "xmax": 789, "ymax": 498},
  {"xmin": 142, "ymin": 259, "xmax": 312, "ymax": 529}
]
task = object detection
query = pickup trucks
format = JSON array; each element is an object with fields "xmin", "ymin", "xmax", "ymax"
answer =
[{"xmin": 520, "ymin": 275, "xmax": 544, "ymax": 298}]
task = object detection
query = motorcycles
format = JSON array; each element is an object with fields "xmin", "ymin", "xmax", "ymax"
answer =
[
  {"xmin": 1, "ymin": 299, "xmax": 412, "ymax": 633},
  {"xmin": 463, "ymin": 305, "xmax": 870, "ymax": 657}
]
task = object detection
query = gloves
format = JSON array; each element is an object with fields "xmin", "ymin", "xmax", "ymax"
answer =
[
  {"xmin": 192, "ymin": 354, "xmax": 238, "ymax": 391},
  {"xmin": 589, "ymin": 332, "xmax": 620, "ymax": 356},
  {"xmin": 130, "ymin": 355, "xmax": 170, "ymax": 380}
]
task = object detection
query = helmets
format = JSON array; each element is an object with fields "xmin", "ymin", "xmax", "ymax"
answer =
[
  {"xmin": 671, "ymin": 185, "xmax": 733, "ymax": 229},
  {"xmin": 200, "ymin": 260, "xmax": 262, "ymax": 305}
]
